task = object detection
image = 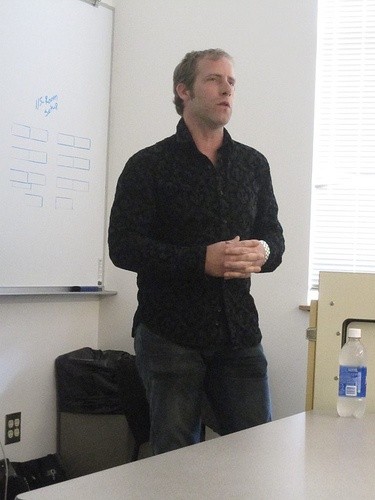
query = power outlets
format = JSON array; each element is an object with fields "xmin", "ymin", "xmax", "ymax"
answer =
[{"xmin": 5, "ymin": 412, "xmax": 21, "ymax": 446}]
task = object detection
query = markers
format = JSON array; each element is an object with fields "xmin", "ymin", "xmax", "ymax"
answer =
[{"xmin": 68, "ymin": 286, "xmax": 101, "ymax": 292}]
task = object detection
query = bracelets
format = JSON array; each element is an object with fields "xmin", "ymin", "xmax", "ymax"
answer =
[{"xmin": 259, "ymin": 241, "xmax": 271, "ymax": 265}]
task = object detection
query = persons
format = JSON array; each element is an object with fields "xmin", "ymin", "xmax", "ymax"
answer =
[{"xmin": 107, "ymin": 47, "xmax": 286, "ymax": 455}]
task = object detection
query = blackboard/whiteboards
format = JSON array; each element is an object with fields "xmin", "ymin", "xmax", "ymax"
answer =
[{"xmin": 0, "ymin": 0, "xmax": 117, "ymax": 297}]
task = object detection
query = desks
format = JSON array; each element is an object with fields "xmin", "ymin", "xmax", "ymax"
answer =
[{"xmin": 16, "ymin": 412, "xmax": 375, "ymax": 500}]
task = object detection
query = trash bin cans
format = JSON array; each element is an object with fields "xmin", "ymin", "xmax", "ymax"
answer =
[{"xmin": 53, "ymin": 347, "xmax": 148, "ymax": 480}]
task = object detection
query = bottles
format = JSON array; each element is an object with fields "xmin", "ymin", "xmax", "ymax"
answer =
[{"xmin": 337, "ymin": 329, "xmax": 367, "ymax": 418}]
